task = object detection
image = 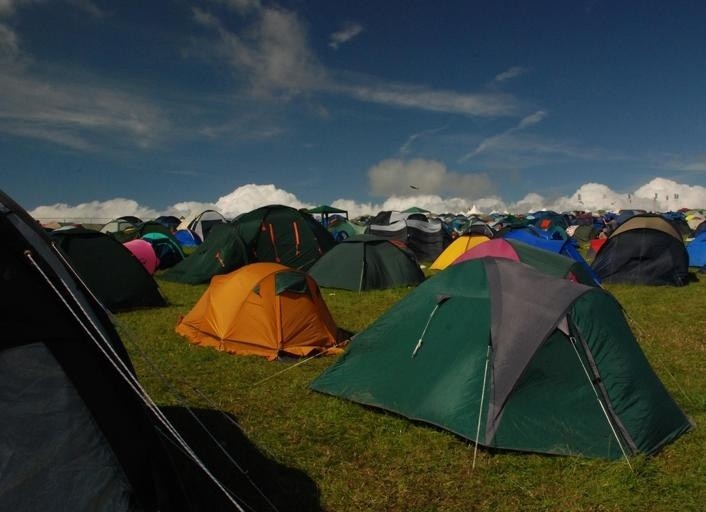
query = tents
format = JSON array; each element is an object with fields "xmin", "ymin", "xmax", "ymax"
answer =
[
  {"xmin": 174, "ymin": 229, "xmax": 203, "ymax": 249},
  {"xmin": 364, "ymin": 210, "xmax": 452, "ymax": 262},
  {"xmin": 590, "ymin": 213, "xmax": 690, "ymax": 287},
  {"xmin": 686, "ymin": 231, "xmax": 705, "ymax": 268},
  {"xmin": 438, "ymin": 204, "xmax": 592, "ymax": 240},
  {"xmin": 0, "ymin": 188, "xmax": 279, "ymax": 512},
  {"xmin": 156, "ymin": 204, "xmax": 334, "ymax": 284},
  {"xmin": 137, "ymin": 221, "xmax": 170, "ymax": 239},
  {"xmin": 319, "ymin": 214, "xmax": 376, "ymax": 241},
  {"xmin": 307, "ymin": 255, "xmax": 693, "ymax": 464},
  {"xmin": 177, "ymin": 208, "xmax": 228, "ymax": 231},
  {"xmin": 174, "ymin": 261, "xmax": 348, "ymax": 361},
  {"xmin": 587, "ymin": 232, "xmax": 608, "ymax": 258},
  {"xmin": 661, "ymin": 206, "xmax": 706, "ymax": 237},
  {"xmin": 429, "ymin": 232, "xmax": 491, "ymax": 271},
  {"xmin": 307, "ymin": 234, "xmax": 427, "ymax": 292},
  {"xmin": 503, "ymin": 229, "xmax": 600, "ymax": 287},
  {"xmin": 49, "ymin": 226, "xmax": 171, "ymax": 312},
  {"xmin": 142, "ymin": 232, "xmax": 185, "ymax": 272},
  {"xmin": 117, "ymin": 215, "xmax": 143, "ymax": 226},
  {"xmin": 100, "ymin": 218, "xmax": 135, "ymax": 234},
  {"xmin": 120, "ymin": 239, "xmax": 161, "ymax": 275},
  {"xmin": 153, "ymin": 215, "xmax": 181, "ymax": 231},
  {"xmin": 447, "ymin": 237, "xmax": 596, "ymax": 291}
]
